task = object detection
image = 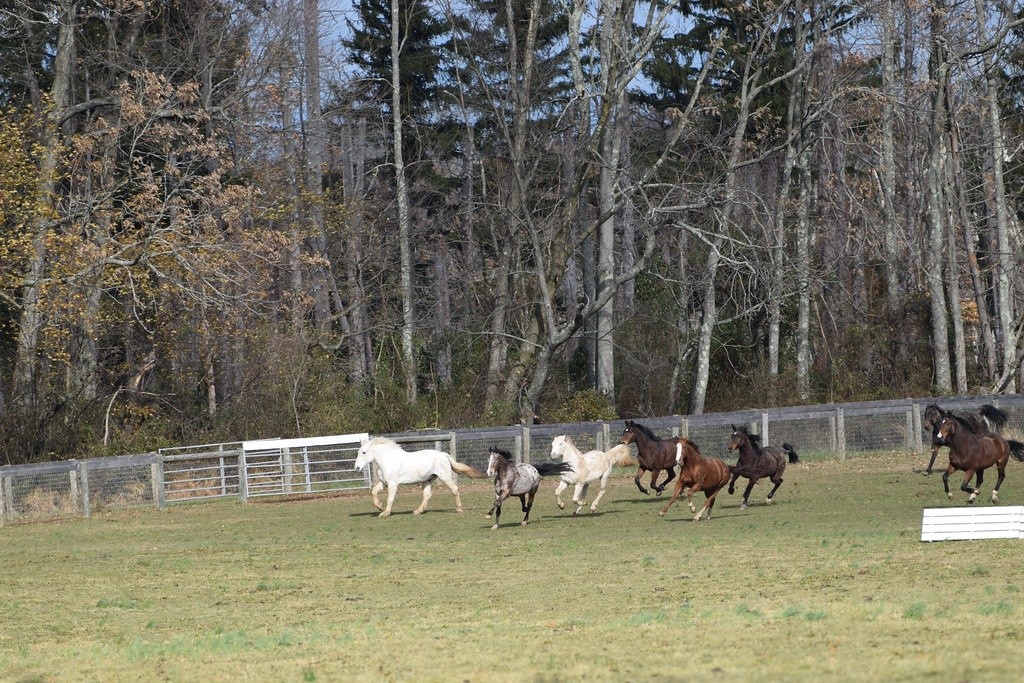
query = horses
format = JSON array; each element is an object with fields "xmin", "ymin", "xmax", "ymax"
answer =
[
  {"xmin": 351, "ymin": 433, "xmax": 482, "ymax": 518},
  {"xmin": 482, "ymin": 419, "xmax": 799, "ymax": 530},
  {"xmin": 922, "ymin": 401, "xmax": 1024, "ymax": 505}
]
